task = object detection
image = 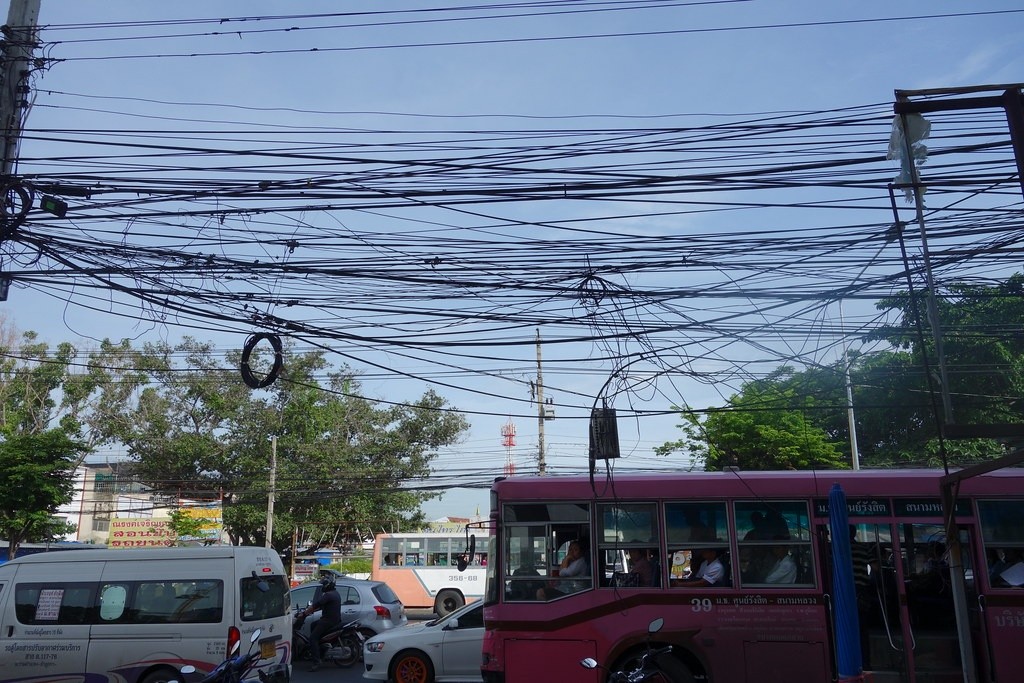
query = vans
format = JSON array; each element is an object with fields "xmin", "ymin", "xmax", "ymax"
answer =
[{"xmin": 1, "ymin": 545, "xmax": 293, "ymax": 682}]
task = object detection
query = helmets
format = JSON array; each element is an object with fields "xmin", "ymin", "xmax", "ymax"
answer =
[{"xmin": 319, "ymin": 575, "xmax": 336, "ymax": 593}]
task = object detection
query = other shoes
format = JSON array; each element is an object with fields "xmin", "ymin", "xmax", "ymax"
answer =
[{"xmin": 312, "ymin": 663, "xmax": 320, "ymax": 672}]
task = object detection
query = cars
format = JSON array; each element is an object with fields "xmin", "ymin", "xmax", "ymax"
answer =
[
  {"xmin": 288, "ymin": 578, "xmax": 409, "ymax": 663},
  {"xmin": 321, "ymin": 568, "xmax": 347, "ymax": 578},
  {"xmin": 360, "ymin": 595, "xmax": 486, "ymax": 683}
]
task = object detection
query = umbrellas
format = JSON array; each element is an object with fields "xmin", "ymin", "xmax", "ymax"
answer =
[{"xmin": 824, "ymin": 481, "xmax": 873, "ymax": 683}]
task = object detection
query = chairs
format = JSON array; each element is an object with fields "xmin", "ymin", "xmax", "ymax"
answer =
[{"xmin": 651, "ymin": 562, "xmax": 660, "ymax": 588}]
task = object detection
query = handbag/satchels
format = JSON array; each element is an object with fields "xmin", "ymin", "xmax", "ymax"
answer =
[{"xmin": 611, "ymin": 571, "xmax": 639, "ymax": 587}]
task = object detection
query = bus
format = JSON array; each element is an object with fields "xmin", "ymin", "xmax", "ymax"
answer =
[
  {"xmin": 455, "ymin": 465, "xmax": 1024, "ymax": 683},
  {"xmin": 372, "ymin": 529, "xmax": 629, "ymax": 619}
]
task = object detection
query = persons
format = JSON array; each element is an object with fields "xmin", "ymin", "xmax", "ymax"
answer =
[
  {"xmin": 670, "ymin": 510, "xmax": 800, "ymax": 587},
  {"xmin": 829, "ymin": 524, "xmax": 876, "ymax": 672},
  {"xmin": 297, "ymin": 575, "xmax": 341, "ymax": 672},
  {"xmin": 627, "ymin": 536, "xmax": 660, "ymax": 587},
  {"xmin": 536, "ymin": 541, "xmax": 589, "ymax": 601}
]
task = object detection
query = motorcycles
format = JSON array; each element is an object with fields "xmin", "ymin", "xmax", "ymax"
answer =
[{"xmin": 292, "ymin": 598, "xmax": 366, "ymax": 670}]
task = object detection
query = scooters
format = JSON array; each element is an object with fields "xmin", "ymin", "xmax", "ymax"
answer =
[{"xmin": 181, "ymin": 626, "xmax": 268, "ymax": 683}]
